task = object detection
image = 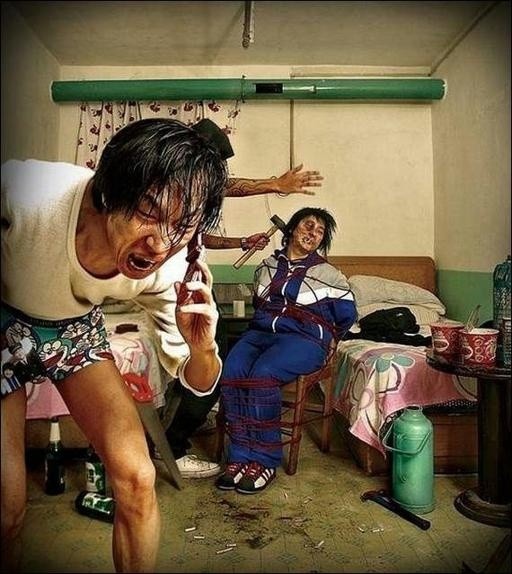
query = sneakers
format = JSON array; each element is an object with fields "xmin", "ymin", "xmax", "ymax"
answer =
[
  {"xmin": 161, "ymin": 456, "xmax": 220, "ymax": 479},
  {"xmin": 235, "ymin": 462, "xmax": 276, "ymax": 494},
  {"xmin": 216, "ymin": 462, "xmax": 249, "ymax": 489},
  {"xmin": 193, "ymin": 418, "xmax": 217, "ymax": 435}
]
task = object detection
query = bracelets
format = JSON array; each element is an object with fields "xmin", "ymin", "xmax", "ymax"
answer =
[{"xmin": 240, "ymin": 237, "xmax": 247, "ymax": 251}]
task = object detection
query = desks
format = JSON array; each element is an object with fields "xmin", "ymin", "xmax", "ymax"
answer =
[{"xmin": 426, "ymin": 352, "xmax": 512, "ymax": 530}]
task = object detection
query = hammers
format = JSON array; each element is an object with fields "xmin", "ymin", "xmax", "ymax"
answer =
[
  {"xmin": 360, "ymin": 487, "xmax": 430, "ymax": 530},
  {"xmin": 233, "ymin": 215, "xmax": 285, "ymax": 270},
  {"xmin": 462, "ymin": 531, "xmax": 512, "ymax": 574}
]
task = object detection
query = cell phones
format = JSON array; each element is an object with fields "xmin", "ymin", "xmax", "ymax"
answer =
[{"xmin": 173, "ymin": 231, "xmax": 207, "ymax": 305}]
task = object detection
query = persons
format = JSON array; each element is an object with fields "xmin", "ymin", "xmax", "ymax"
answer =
[
  {"xmin": 150, "ymin": 164, "xmax": 326, "ymax": 481},
  {"xmin": 213, "ymin": 205, "xmax": 358, "ymax": 495},
  {"xmin": 0, "ymin": 116, "xmax": 223, "ymax": 574}
]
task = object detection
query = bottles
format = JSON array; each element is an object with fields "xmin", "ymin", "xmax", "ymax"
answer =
[
  {"xmin": 42, "ymin": 416, "xmax": 66, "ymax": 497},
  {"xmin": 73, "ymin": 443, "xmax": 116, "ymax": 524},
  {"xmin": 381, "ymin": 404, "xmax": 436, "ymax": 516}
]
